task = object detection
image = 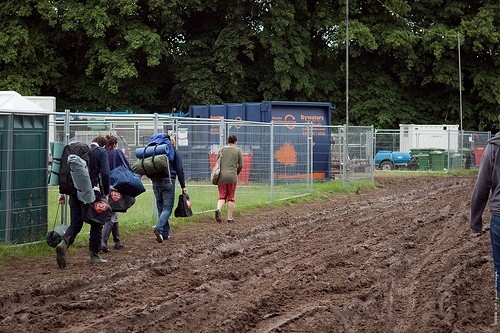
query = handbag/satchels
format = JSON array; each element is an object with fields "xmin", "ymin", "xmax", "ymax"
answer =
[
  {"xmin": 107, "ymin": 191, "xmax": 137, "ymax": 214},
  {"xmin": 108, "ymin": 148, "xmax": 146, "ymax": 198},
  {"xmin": 81, "ymin": 198, "xmax": 113, "ymax": 226},
  {"xmin": 174, "ymin": 191, "xmax": 193, "ymax": 217},
  {"xmin": 45, "ymin": 202, "xmax": 69, "ymax": 248},
  {"xmin": 210, "ymin": 147, "xmax": 221, "ymax": 185}
]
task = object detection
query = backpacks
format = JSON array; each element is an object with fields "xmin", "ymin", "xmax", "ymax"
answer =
[
  {"xmin": 130, "ymin": 132, "xmax": 175, "ymax": 176},
  {"xmin": 58, "ymin": 141, "xmax": 100, "ymax": 197}
]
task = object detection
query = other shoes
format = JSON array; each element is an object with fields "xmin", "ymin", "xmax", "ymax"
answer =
[
  {"xmin": 89, "ymin": 251, "xmax": 108, "ymax": 264},
  {"xmin": 113, "ymin": 242, "xmax": 124, "ymax": 250},
  {"xmin": 56, "ymin": 239, "xmax": 69, "ymax": 270},
  {"xmin": 153, "ymin": 228, "xmax": 171, "ymax": 243},
  {"xmin": 227, "ymin": 217, "xmax": 235, "ymax": 223},
  {"xmin": 100, "ymin": 244, "xmax": 110, "ymax": 254},
  {"xmin": 214, "ymin": 209, "xmax": 222, "ymax": 223}
]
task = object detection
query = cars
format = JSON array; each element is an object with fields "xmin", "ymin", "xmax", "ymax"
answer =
[{"xmin": 374, "ymin": 150, "xmax": 410, "ymax": 171}]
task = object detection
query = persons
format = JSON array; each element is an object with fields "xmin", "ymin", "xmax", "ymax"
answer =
[
  {"xmin": 98, "ymin": 135, "xmax": 134, "ymax": 252},
  {"xmin": 56, "ymin": 137, "xmax": 111, "ymax": 267},
  {"xmin": 215, "ymin": 135, "xmax": 242, "ymax": 223},
  {"xmin": 148, "ymin": 137, "xmax": 187, "ymax": 243},
  {"xmin": 470, "ymin": 130, "xmax": 500, "ymax": 291}
]
